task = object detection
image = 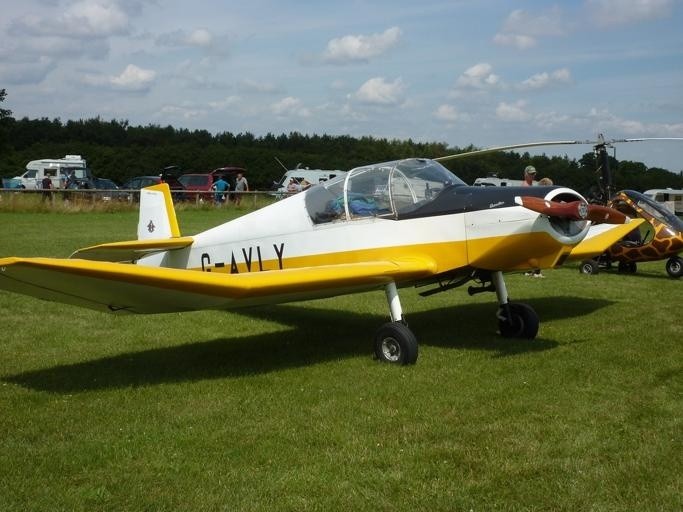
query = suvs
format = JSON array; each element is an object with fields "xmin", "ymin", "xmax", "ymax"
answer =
[
  {"xmin": 178, "ymin": 166, "xmax": 248, "ymax": 202},
  {"xmin": 124, "ymin": 165, "xmax": 187, "ymax": 204}
]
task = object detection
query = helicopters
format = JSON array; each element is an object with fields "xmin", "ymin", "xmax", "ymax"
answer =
[{"xmin": 430, "ymin": 133, "xmax": 683, "ymax": 279}]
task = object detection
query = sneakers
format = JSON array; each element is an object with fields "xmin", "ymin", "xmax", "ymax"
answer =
[{"xmin": 533, "ymin": 274, "xmax": 545, "ymax": 279}]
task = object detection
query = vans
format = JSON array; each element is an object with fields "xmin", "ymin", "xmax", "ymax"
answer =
[
  {"xmin": 471, "ymin": 176, "xmax": 541, "ymax": 187},
  {"xmin": 637, "ymin": 187, "xmax": 683, "ymax": 215},
  {"xmin": 277, "ymin": 167, "xmax": 348, "ymax": 192}
]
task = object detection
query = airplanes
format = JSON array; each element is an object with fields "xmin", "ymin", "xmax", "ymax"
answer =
[{"xmin": 0, "ymin": 150, "xmax": 645, "ymax": 366}]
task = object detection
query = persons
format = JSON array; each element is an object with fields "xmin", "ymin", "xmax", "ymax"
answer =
[
  {"xmin": 349, "ymin": 175, "xmax": 390, "ymax": 215},
  {"xmin": 287, "ymin": 179, "xmax": 297, "ymax": 192},
  {"xmin": 233, "ymin": 173, "xmax": 249, "ymax": 205},
  {"xmin": 524, "ymin": 178, "xmax": 553, "ymax": 279},
  {"xmin": 424, "ymin": 183, "xmax": 432, "ymax": 200},
  {"xmin": 41, "ymin": 173, "xmax": 52, "ymax": 203},
  {"xmin": 521, "ymin": 165, "xmax": 538, "ymax": 187},
  {"xmin": 210, "ymin": 175, "xmax": 230, "ymax": 208},
  {"xmin": 62, "ymin": 178, "xmax": 69, "ymax": 208}
]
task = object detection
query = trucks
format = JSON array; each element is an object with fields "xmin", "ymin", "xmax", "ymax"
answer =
[{"xmin": 13, "ymin": 155, "xmax": 88, "ymax": 192}]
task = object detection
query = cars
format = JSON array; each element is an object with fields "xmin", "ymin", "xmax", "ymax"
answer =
[{"xmin": 61, "ymin": 177, "xmax": 130, "ymax": 206}]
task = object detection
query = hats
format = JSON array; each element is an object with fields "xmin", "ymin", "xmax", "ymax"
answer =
[{"xmin": 524, "ymin": 166, "xmax": 536, "ymax": 174}]
